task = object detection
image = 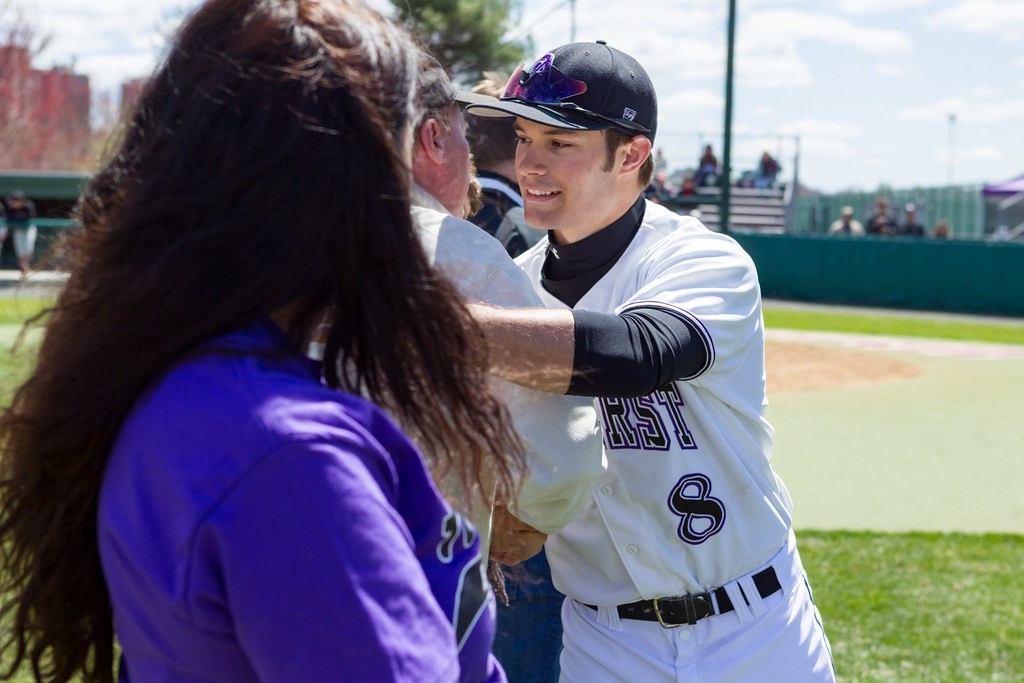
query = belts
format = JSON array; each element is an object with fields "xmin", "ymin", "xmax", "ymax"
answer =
[{"xmin": 583, "ymin": 566, "xmax": 782, "ymax": 629}]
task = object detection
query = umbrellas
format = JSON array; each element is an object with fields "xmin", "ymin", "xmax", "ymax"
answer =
[{"xmin": 983, "ymin": 176, "xmax": 1024, "ymax": 199}]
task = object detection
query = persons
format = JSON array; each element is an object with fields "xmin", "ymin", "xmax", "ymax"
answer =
[
  {"xmin": 308, "ymin": 39, "xmax": 838, "ymax": 683},
  {"xmin": 736, "ymin": 151, "xmax": 782, "ymax": 189},
  {"xmin": 643, "ymin": 144, "xmax": 722, "ymax": 222},
  {"xmin": 5, "ymin": 192, "xmax": 37, "ymax": 280},
  {"xmin": 1, "ymin": 0, "xmax": 528, "ymax": 683},
  {"xmin": 829, "ymin": 195, "xmax": 949, "ymax": 237}
]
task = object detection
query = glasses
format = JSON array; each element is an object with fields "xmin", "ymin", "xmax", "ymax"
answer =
[{"xmin": 500, "ymin": 64, "xmax": 652, "ymax": 133}]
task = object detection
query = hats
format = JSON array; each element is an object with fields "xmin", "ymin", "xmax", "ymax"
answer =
[
  {"xmin": 410, "ymin": 49, "xmax": 500, "ymax": 115},
  {"xmin": 466, "ymin": 41, "xmax": 658, "ymax": 145}
]
